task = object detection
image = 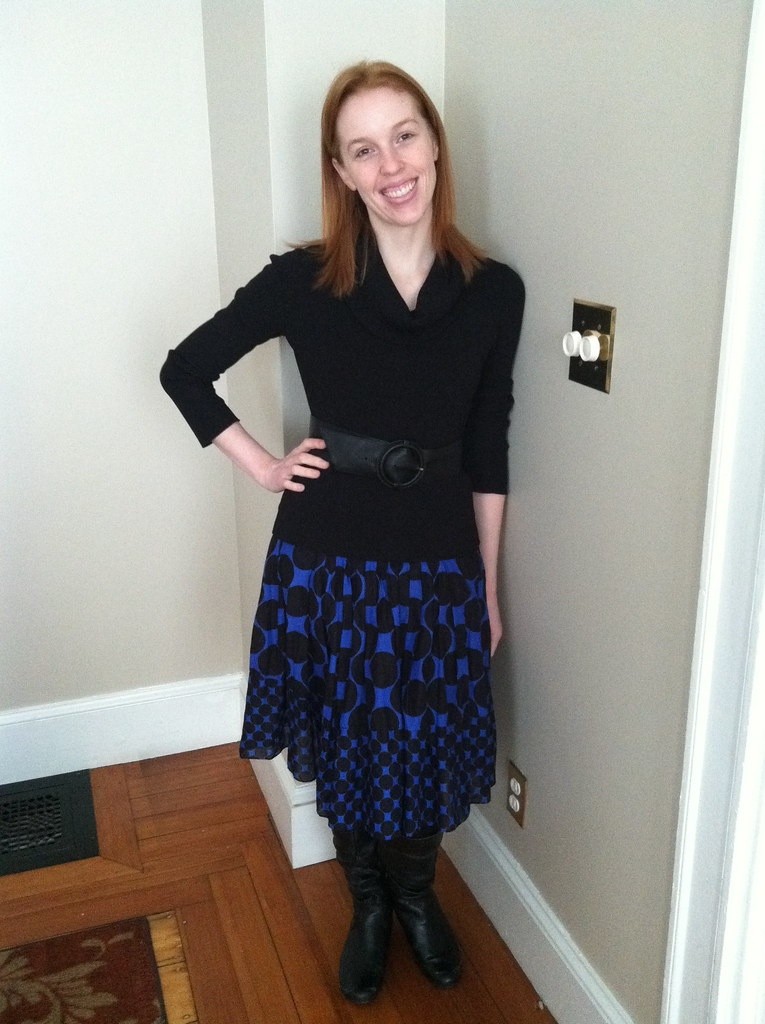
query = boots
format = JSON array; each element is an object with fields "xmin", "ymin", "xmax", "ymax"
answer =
[
  {"xmin": 389, "ymin": 826, "xmax": 461, "ymax": 990},
  {"xmin": 333, "ymin": 837, "xmax": 390, "ymax": 1003}
]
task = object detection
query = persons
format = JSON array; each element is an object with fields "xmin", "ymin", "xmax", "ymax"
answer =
[{"xmin": 160, "ymin": 60, "xmax": 525, "ymax": 1005}]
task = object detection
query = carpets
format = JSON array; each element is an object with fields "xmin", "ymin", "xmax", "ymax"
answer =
[
  {"xmin": 0, "ymin": 769, "xmax": 99, "ymax": 877},
  {"xmin": 0, "ymin": 916, "xmax": 169, "ymax": 1024}
]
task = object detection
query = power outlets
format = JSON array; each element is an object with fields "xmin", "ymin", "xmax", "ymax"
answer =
[{"xmin": 506, "ymin": 760, "xmax": 528, "ymax": 830}]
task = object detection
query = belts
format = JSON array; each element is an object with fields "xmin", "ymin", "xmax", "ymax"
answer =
[{"xmin": 309, "ymin": 417, "xmax": 465, "ymax": 489}]
